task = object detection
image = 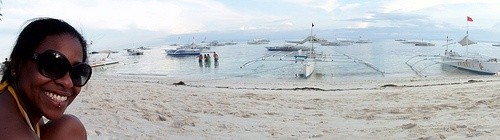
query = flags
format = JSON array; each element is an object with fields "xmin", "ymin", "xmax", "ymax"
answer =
[
  {"xmin": 312, "ymin": 23, "xmax": 314, "ymax": 27},
  {"xmin": 467, "ymin": 16, "xmax": 473, "ymax": 22}
]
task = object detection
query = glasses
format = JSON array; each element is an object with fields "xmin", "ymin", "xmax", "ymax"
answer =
[{"xmin": 29, "ymin": 49, "xmax": 92, "ymax": 87}]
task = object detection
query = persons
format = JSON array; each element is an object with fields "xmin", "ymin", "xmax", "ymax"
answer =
[
  {"xmin": 204, "ymin": 54, "xmax": 208, "ymax": 64},
  {"xmin": 0, "ymin": 17, "xmax": 92, "ymax": 140},
  {"xmin": 213, "ymin": 52, "xmax": 219, "ymax": 62},
  {"xmin": 2, "ymin": 56, "xmax": 10, "ymax": 70},
  {"xmin": 207, "ymin": 54, "xmax": 211, "ymax": 64},
  {"xmin": 196, "ymin": 54, "xmax": 203, "ymax": 62}
]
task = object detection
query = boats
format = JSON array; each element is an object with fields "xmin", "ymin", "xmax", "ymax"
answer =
[
  {"xmin": 441, "ymin": 34, "xmax": 500, "ymax": 74},
  {"xmin": 295, "ymin": 22, "xmax": 316, "ymax": 78},
  {"xmin": 397, "ymin": 39, "xmax": 434, "ymax": 46},
  {"xmin": 86, "ymin": 35, "xmax": 271, "ymax": 69},
  {"xmin": 337, "ymin": 36, "xmax": 371, "ymax": 44},
  {"xmin": 266, "ymin": 45, "xmax": 317, "ymax": 51}
]
